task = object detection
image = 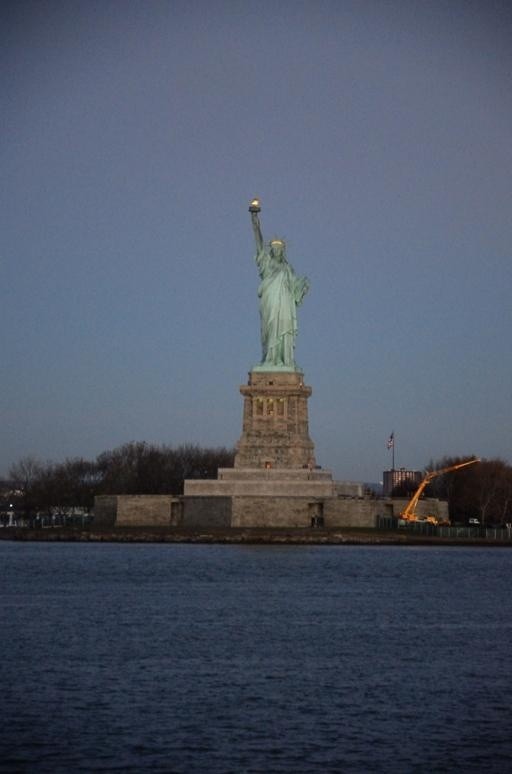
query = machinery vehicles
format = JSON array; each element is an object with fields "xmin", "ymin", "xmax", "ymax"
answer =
[{"xmin": 398, "ymin": 458, "xmax": 481, "ymax": 526}]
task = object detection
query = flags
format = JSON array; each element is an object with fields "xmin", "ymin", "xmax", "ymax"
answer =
[{"xmin": 383, "ymin": 433, "xmax": 393, "ymax": 449}]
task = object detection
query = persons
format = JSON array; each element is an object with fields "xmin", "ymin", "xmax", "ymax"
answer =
[
  {"xmin": 311, "ymin": 512, "xmax": 316, "ymax": 527},
  {"xmin": 248, "ymin": 199, "xmax": 310, "ymax": 373}
]
